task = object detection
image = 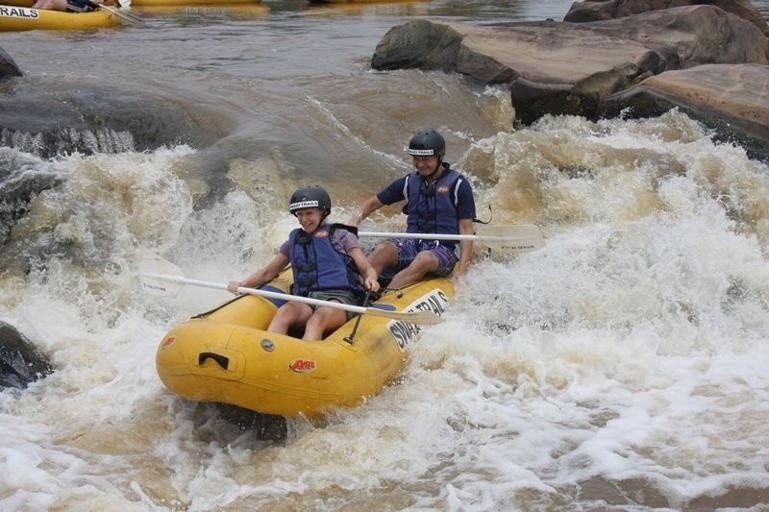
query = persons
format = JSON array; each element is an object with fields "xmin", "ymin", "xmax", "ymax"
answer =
[
  {"xmin": 28, "ymin": 0, "xmax": 104, "ymax": 12},
  {"xmin": 346, "ymin": 128, "xmax": 479, "ymax": 296},
  {"xmin": 224, "ymin": 184, "xmax": 382, "ymax": 343}
]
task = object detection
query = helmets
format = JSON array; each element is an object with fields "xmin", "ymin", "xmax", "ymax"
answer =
[
  {"xmin": 407, "ymin": 129, "xmax": 445, "ymax": 157},
  {"xmin": 289, "ymin": 185, "xmax": 331, "ymax": 215}
]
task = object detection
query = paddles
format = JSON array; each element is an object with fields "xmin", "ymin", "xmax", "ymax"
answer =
[
  {"xmin": 265, "ymin": 224, "xmax": 542, "ymax": 253},
  {"xmin": 138, "ymin": 256, "xmax": 443, "ymax": 324}
]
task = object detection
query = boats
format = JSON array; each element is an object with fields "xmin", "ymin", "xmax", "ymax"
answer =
[
  {"xmin": 0, "ymin": 0, "xmax": 272, "ymax": 29},
  {"xmin": 155, "ymin": 237, "xmax": 494, "ymax": 418}
]
